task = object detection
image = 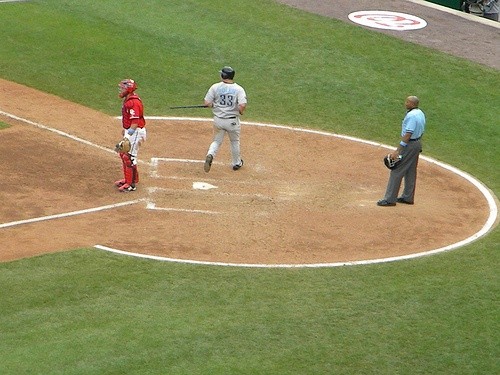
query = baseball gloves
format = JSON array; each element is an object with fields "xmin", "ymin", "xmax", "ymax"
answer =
[
  {"xmin": 384, "ymin": 153, "xmax": 402, "ymax": 170},
  {"xmin": 114, "ymin": 137, "xmax": 131, "ymax": 154}
]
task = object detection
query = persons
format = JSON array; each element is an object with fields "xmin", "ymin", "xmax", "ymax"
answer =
[
  {"xmin": 202, "ymin": 67, "xmax": 247, "ymax": 172},
  {"xmin": 115, "ymin": 79, "xmax": 147, "ymax": 192},
  {"xmin": 376, "ymin": 95, "xmax": 426, "ymax": 207}
]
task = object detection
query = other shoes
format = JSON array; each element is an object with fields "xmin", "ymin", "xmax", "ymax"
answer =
[
  {"xmin": 233, "ymin": 159, "xmax": 243, "ymax": 170},
  {"xmin": 397, "ymin": 197, "xmax": 414, "ymax": 205},
  {"xmin": 204, "ymin": 154, "xmax": 213, "ymax": 173},
  {"xmin": 126, "ymin": 184, "xmax": 136, "ymax": 190},
  {"xmin": 377, "ymin": 199, "xmax": 396, "ymax": 206}
]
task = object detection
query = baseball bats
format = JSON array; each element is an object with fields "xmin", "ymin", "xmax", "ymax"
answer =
[{"xmin": 169, "ymin": 105, "xmax": 210, "ymax": 110}]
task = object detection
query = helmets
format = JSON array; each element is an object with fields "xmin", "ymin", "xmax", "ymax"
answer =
[
  {"xmin": 384, "ymin": 154, "xmax": 399, "ymax": 170},
  {"xmin": 219, "ymin": 66, "xmax": 235, "ymax": 80},
  {"xmin": 117, "ymin": 79, "xmax": 137, "ymax": 98}
]
